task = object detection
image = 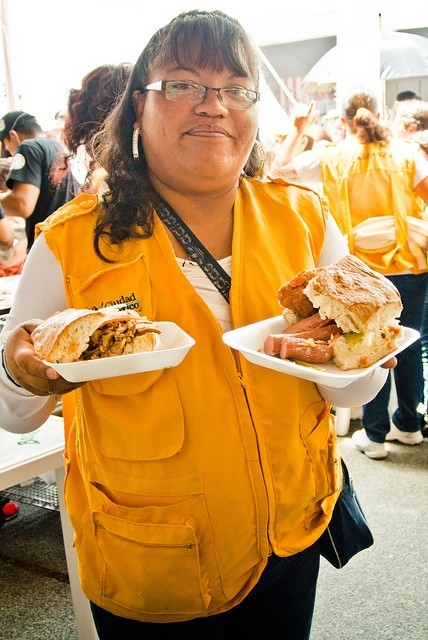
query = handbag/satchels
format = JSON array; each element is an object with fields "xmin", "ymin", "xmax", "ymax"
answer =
[{"xmin": 321, "ymin": 458, "xmax": 374, "ymax": 569}]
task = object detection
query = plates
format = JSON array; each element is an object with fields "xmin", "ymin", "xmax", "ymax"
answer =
[
  {"xmin": 221, "ymin": 314, "xmax": 421, "ymax": 389},
  {"xmin": 42, "ymin": 320, "xmax": 197, "ymax": 384}
]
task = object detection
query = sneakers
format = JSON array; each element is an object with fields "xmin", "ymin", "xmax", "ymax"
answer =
[
  {"xmin": 386, "ymin": 418, "xmax": 424, "ymax": 445},
  {"xmin": 352, "ymin": 428, "xmax": 388, "ymax": 459}
]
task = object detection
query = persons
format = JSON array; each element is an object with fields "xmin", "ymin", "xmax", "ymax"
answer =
[
  {"xmin": 270, "ymin": 91, "xmax": 428, "ymax": 180},
  {"xmin": 0, "ymin": 112, "xmax": 81, "ymax": 251},
  {"xmin": 0, "ymin": 9, "xmax": 398, "ymax": 640},
  {"xmin": 270, "ymin": 93, "xmax": 428, "ymax": 457},
  {"xmin": 0, "ymin": 204, "xmax": 18, "ymax": 250}
]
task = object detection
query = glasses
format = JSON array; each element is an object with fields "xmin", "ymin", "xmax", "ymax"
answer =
[{"xmin": 142, "ymin": 80, "xmax": 262, "ymax": 110}]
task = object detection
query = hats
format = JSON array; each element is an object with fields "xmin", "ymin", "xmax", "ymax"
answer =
[{"xmin": 0, "ymin": 112, "xmax": 27, "ymax": 139}]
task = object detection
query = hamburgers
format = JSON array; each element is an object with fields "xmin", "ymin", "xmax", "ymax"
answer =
[
  {"xmin": 263, "ymin": 254, "xmax": 404, "ymax": 372},
  {"xmin": 29, "ymin": 307, "xmax": 162, "ymax": 365}
]
